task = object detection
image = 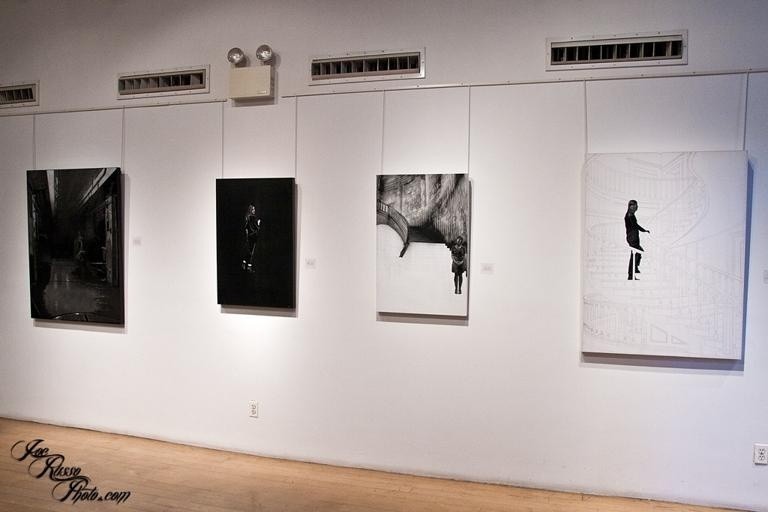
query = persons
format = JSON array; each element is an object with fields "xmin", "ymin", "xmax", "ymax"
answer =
[
  {"xmin": 242, "ymin": 204, "xmax": 260, "ymax": 266},
  {"xmin": 623, "ymin": 199, "xmax": 650, "ymax": 281},
  {"xmin": 450, "ymin": 236, "xmax": 466, "ymax": 293}
]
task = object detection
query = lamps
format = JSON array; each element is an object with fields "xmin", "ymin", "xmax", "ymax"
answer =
[{"xmin": 224, "ymin": 42, "xmax": 275, "ymax": 104}]
